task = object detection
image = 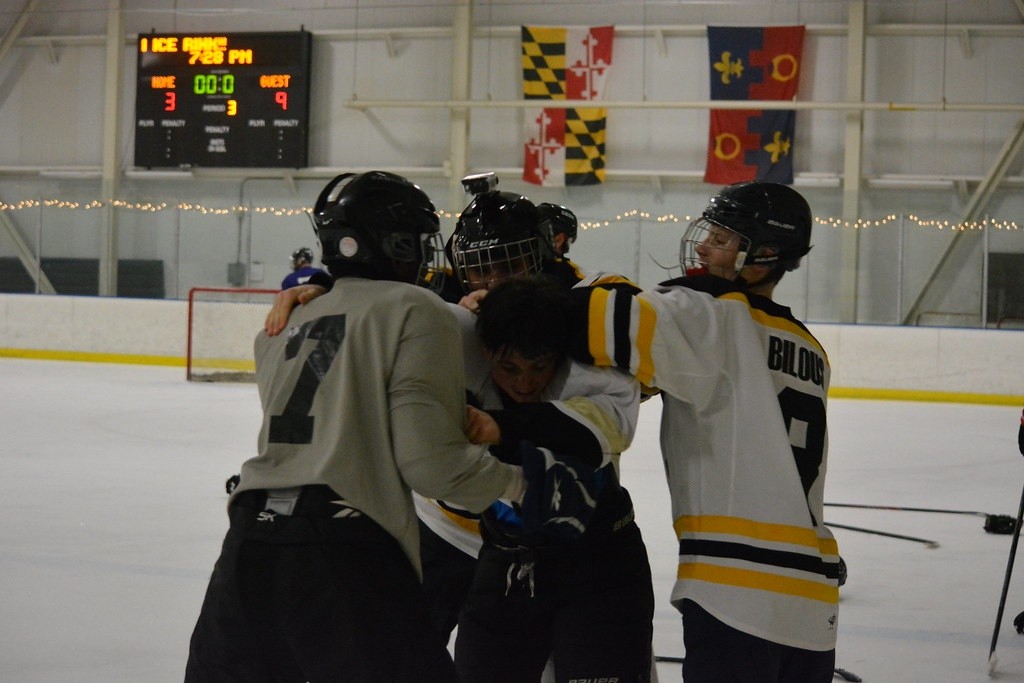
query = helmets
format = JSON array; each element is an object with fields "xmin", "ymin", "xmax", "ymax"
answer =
[
  {"xmin": 536, "ymin": 202, "xmax": 578, "ymax": 245},
  {"xmin": 679, "ymin": 181, "xmax": 816, "ymax": 281},
  {"xmin": 312, "ymin": 171, "xmax": 444, "ymax": 293},
  {"xmin": 292, "ymin": 247, "xmax": 314, "ymax": 267},
  {"xmin": 445, "ymin": 191, "xmax": 552, "ymax": 296}
]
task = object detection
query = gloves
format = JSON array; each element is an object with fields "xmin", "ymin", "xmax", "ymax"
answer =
[{"xmin": 482, "ymin": 446, "xmax": 597, "ymax": 556}]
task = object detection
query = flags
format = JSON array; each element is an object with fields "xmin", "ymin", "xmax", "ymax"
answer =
[
  {"xmin": 704, "ymin": 25, "xmax": 806, "ymax": 185},
  {"xmin": 521, "ymin": 24, "xmax": 614, "ymax": 187}
]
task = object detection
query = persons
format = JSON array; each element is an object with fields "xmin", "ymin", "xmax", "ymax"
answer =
[
  {"xmin": 184, "ymin": 171, "xmax": 848, "ymax": 683},
  {"xmin": 282, "ymin": 248, "xmax": 330, "ymax": 291}
]
